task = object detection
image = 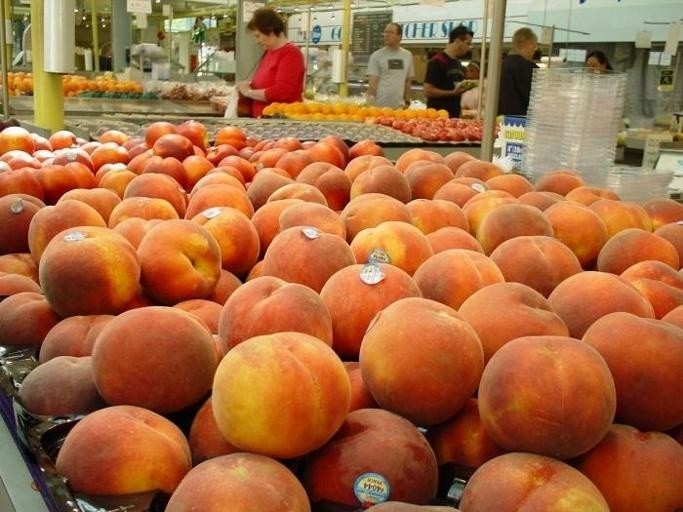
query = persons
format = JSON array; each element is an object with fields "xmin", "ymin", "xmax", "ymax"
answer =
[
  {"xmin": 422, "ymin": 24, "xmax": 473, "ymax": 119},
  {"xmin": 465, "ymin": 62, "xmax": 480, "ymax": 80},
  {"xmin": 365, "ymin": 22, "xmax": 415, "ymax": 108},
  {"xmin": 208, "ymin": 5, "xmax": 305, "ymax": 118},
  {"xmin": 496, "ymin": 26, "xmax": 540, "ymax": 116},
  {"xmin": 584, "ymin": 50, "xmax": 612, "ymax": 72}
]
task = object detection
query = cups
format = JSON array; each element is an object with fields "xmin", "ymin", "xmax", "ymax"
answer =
[{"xmin": 151, "ymin": 62, "xmax": 170, "ymax": 81}]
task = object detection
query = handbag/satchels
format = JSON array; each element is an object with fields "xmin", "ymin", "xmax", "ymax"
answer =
[{"xmin": 235, "ymin": 84, "xmax": 253, "ymax": 117}]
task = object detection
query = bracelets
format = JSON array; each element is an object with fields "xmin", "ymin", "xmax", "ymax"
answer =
[{"xmin": 247, "ymin": 89, "xmax": 252, "ymax": 99}]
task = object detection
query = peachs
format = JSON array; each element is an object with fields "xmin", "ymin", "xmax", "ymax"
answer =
[{"xmin": 1, "ymin": 119, "xmax": 683, "ymax": 512}]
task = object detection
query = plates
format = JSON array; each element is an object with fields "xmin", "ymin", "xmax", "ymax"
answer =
[{"xmin": 523, "ymin": 65, "xmax": 674, "ymax": 203}]
